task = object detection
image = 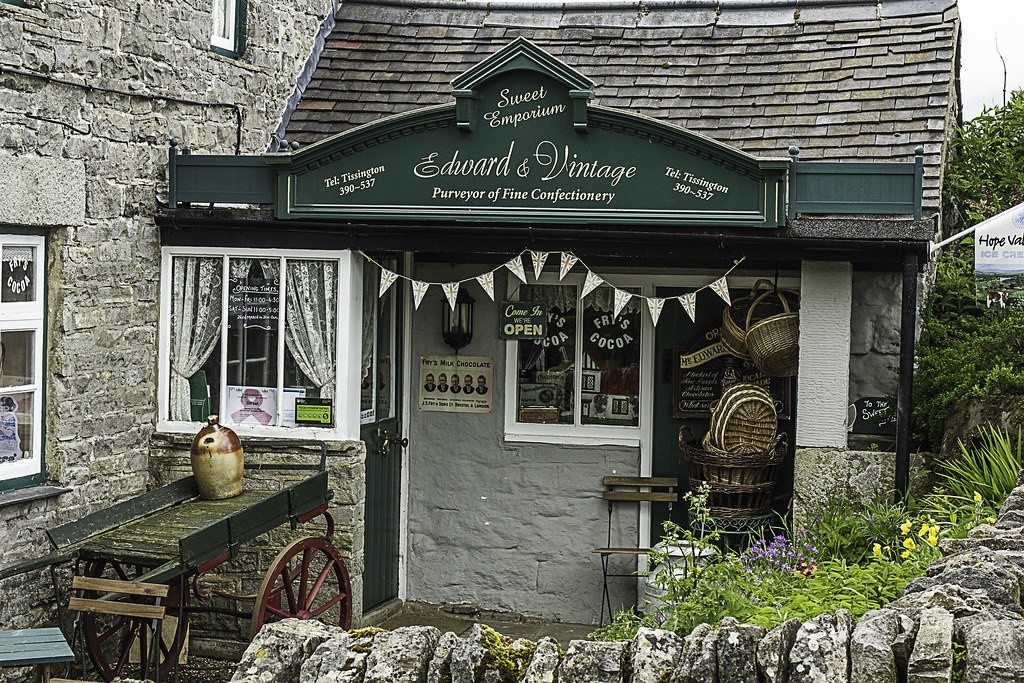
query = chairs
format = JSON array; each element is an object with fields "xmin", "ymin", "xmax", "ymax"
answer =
[
  {"xmin": 50, "ymin": 575, "xmax": 169, "ymax": 683},
  {"xmin": 590, "ymin": 475, "xmax": 678, "ymax": 631}
]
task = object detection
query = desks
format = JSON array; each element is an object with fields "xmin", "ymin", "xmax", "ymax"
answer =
[{"xmin": 0, "ymin": 627, "xmax": 76, "ymax": 683}]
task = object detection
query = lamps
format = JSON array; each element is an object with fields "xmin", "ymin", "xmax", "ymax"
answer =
[{"xmin": 440, "ymin": 288, "xmax": 476, "ymax": 354}]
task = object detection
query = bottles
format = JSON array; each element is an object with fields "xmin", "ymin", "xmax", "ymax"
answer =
[
  {"xmin": 644, "ymin": 540, "xmax": 717, "ymax": 616},
  {"xmin": 190, "ymin": 415, "xmax": 243, "ymax": 500}
]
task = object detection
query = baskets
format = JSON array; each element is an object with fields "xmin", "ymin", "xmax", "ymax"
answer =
[
  {"xmin": 744, "ymin": 288, "xmax": 800, "ymax": 377},
  {"xmin": 677, "ymin": 382, "xmax": 789, "ymax": 560},
  {"xmin": 719, "ymin": 279, "xmax": 800, "ymax": 360}
]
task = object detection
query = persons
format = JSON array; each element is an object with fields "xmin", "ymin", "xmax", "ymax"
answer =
[
  {"xmin": 463, "ymin": 375, "xmax": 474, "ymax": 394},
  {"xmin": 424, "ymin": 373, "xmax": 436, "ymax": 392},
  {"xmin": 476, "ymin": 375, "xmax": 488, "ymax": 394},
  {"xmin": 450, "ymin": 374, "xmax": 461, "ymax": 393},
  {"xmin": 437, "ymin": 373, "xmax": 449, "ymax": 392}
]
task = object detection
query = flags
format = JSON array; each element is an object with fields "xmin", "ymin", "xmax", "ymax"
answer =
[{"xmin": 975, "ymin": 205, "xmax": 1024, "ymax": 276}]
